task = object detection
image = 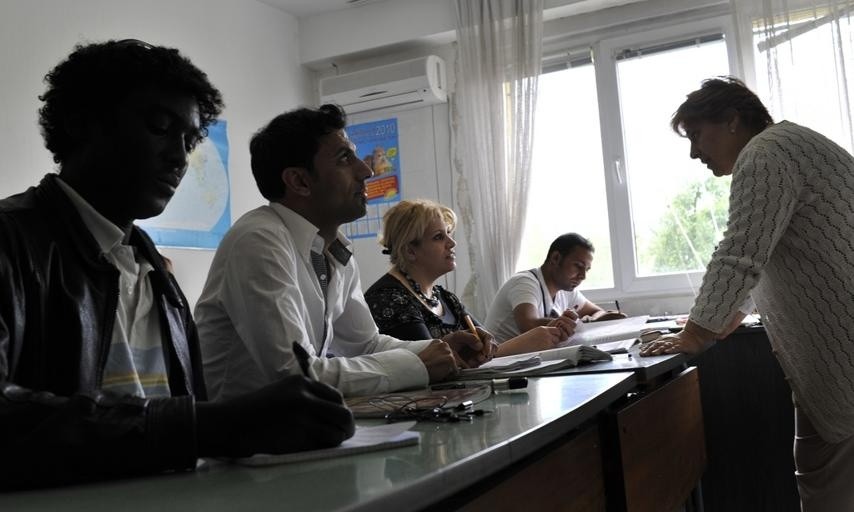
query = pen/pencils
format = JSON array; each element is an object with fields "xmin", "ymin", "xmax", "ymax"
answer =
[
  {"xmin": 464, "ymin": 315, "xmax": 481, "ymax": 341},
  {"xmin": 615, "ymin": 300, "xmax": 621, "ymax": 313},
  {"xmin": 292, "ymin": 341, "xmax": 319, "ymax": 381}
]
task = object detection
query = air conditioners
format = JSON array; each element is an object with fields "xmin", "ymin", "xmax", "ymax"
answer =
[{"xmin": 319, "ymin": 56, "xmax": 450, "ymax": 117}]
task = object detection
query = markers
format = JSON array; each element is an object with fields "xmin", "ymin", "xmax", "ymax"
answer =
[{"xmin": 431, "ymin": 377, "xmax": 528, "ymax": 390}]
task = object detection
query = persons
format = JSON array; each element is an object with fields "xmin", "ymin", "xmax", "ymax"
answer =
[
  {"xmin": 637, "ymin": 77, "xmax": 853, "ymax": 512},
  {"xmin": 365, "ymin": 199, "xmax": 575, "ymax": 358},
  {"xmin": 484, "ymin": 232, "xmax": 605, "ymax": 346},
  {"xmin": 195, "ymin": 101, "xmax": 463, "ymax": 403},
  {"xmin": 0, "ymin": 39, "xmax": 355, "ymax": 489}
]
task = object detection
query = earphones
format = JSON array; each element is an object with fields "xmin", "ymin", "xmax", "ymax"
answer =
[
  {"xmin": 444, "ymin": 410, "xmax": 458, "ymax": 421},
  {"xmin": 432, "ymin": 406, "xmax": 445, "ymax": 417}
]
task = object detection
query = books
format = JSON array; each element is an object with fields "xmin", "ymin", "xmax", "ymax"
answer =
[
  {"xmin": 237, "ymin": 420, "xmax": 420, "ymax": 465},
  {"xmin": 460, "ymin": 346, "xmax": 612, "ymax": 379},
  {"xmin": 561, "ymin": 321, "xmax": 646, "ymax": 352},
  {"xmin": 344, "ymin": 384, "xmax": 492, "ymax": 412}
]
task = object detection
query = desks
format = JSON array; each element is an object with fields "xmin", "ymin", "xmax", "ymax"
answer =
[{"xmin": 1, "ymin": 314, "xmax": 797, "ymax": 512}]
